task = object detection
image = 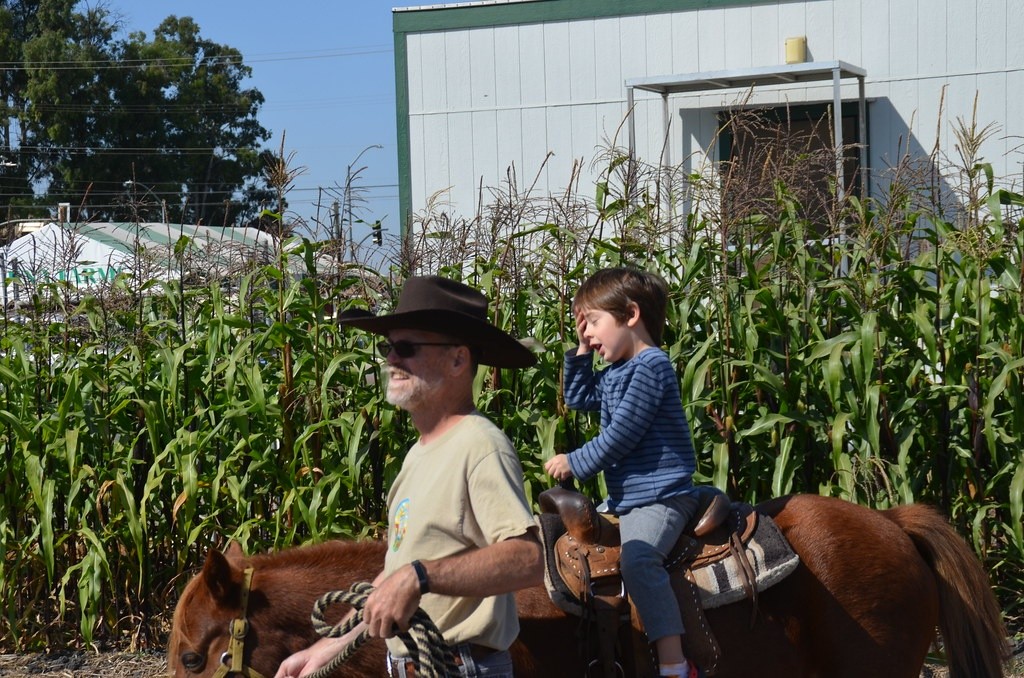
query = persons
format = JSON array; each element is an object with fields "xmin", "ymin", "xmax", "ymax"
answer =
[
  {"xmin": 272, "ymin": 275, "xmax": 547, "ymax": 678},
  {"xmin": 544, "ymin": 267, "xmax": 700, "ymax": 678}
]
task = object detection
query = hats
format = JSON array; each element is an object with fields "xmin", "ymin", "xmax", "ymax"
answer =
[{"xmin": 336, "ymin": 275, "xmax": 538, "ymax": 370}]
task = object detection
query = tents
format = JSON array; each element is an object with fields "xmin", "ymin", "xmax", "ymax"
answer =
[{"xmin": 0, "ymin": 222, "xmax": 388, "ymax": 387}]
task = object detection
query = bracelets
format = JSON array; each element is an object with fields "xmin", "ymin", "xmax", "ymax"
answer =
[{"xmin": 410, "ymin": 560, "xmax": 430, "ymax": 595}]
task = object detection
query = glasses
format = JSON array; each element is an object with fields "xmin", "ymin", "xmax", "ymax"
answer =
[{"xmin": 376, "ymin": 339, "xmax": 457, "ymax": 358}]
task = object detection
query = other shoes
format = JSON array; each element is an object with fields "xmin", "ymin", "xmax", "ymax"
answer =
[{"xmin": 659, "ymin": 659, "xmax": 700, "ymax": 678}]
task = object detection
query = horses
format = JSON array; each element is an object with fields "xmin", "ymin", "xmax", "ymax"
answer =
[{"xmin": 169, "ymin": 490, "xmax": 1013, "ymax": 678}]
